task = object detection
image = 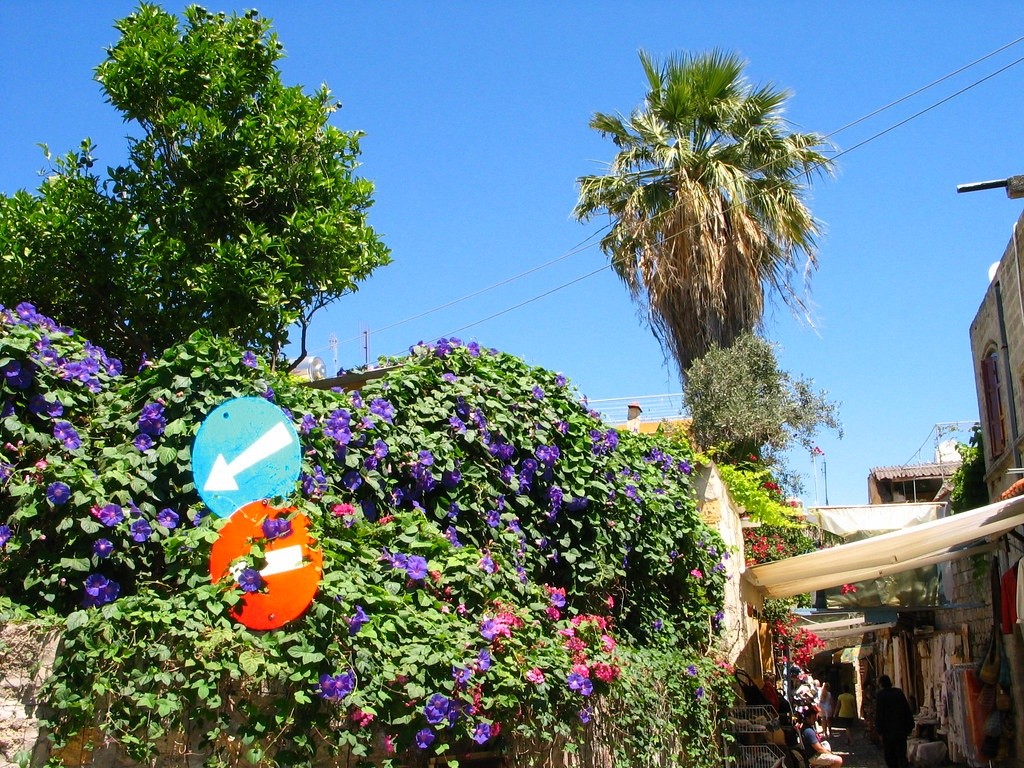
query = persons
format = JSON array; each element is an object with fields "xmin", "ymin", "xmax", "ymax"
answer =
[
  {"xmin": 872, "ymin": 675, "xmax": 916, "ymax": 768},
  {"xmin": 801, "ymin": 705, "xmax": 843, "ymax": 768},
  {"xmin": 811, "ymin": 679, "xmax": 821, "ymax": 703},
  {"xmin": 818, "ymin": 682, "xmax": 833, "ymax": 739},
  {"xmin": 833, "ymin": 684, "xmax": 858, "ymax": 747}
]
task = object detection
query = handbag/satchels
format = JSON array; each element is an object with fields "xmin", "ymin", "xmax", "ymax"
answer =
[
  {"xmin": 977, "ymin": 626, "xmax": 1016, "ymax": 763},
  {"xmin": 734, "ymin": 669, "xmax": 791, "ymax": 712}
]
